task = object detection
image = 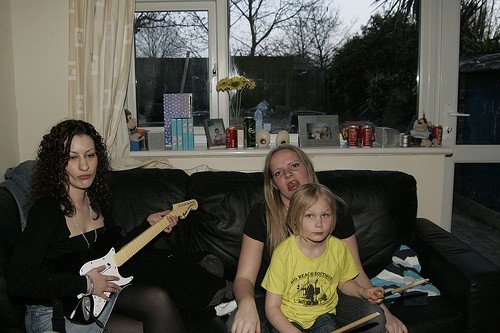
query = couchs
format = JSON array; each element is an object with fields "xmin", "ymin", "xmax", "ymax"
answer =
[{"xmin": 0, "ymin": 168, "xmax": 500, "ymax": 333}]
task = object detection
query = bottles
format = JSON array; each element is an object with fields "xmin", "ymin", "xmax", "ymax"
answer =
[{"xmin": 254, "ymin": 108, "xmax": 262, "ymax": 132}]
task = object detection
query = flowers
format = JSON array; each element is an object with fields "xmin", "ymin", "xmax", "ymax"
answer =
[
  {"xmin": 216, "ymin": 76, "xmax": 256, "ymax": 129},
  {"xmin": 204, "ymin": 119, "xmax": 227, "ymax": 147}
]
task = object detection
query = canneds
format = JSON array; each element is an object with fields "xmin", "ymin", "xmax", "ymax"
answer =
[
  {"xmin": 348, "ymin": 125, "xmax": 359, "ymax": 148},
  {"xmin": 362, "ymin": 125, "xmax": 371, "ymax": 148},
  {"xmin": 225, "ymin": 126, "xmax": 237, "ymax": 149},
  {"xmin": 432, "ymin": 124, "xmax": 442, "ymax": 146},
  {"xmin": 243, "ymin": 117, "xmax": 256, "ymax": 149}
]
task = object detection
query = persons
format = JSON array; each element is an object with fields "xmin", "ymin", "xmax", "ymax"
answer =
[
  {"xmin": 226, "ymin": 145, "xmax": 408, "ymax": 333},
  {"xmin": 214, "ymin": 129, "xmax": 222, "ymax": 144},
  {"xmin": 7, "ymin": 120, "xmax": 186, "ymax": 333},
  {"xmin": 261, "ymin": 183, "xmax": 385, "ymax": 333},
  {"xmin": 310, "ymin": 126, "xmax": 328, "ymax": 138}
]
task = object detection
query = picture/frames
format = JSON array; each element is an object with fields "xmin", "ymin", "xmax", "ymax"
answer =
[{"xmin": 298, "ymin": 115, "xmax": 341, "ymax": 147}]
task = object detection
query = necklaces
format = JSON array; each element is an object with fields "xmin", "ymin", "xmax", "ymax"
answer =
[{"xmin": 72, "ymin": 194, "xmax": 97, "ymax": 248}]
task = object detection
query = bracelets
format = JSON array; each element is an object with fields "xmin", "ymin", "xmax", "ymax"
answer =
[{"xmin": 86, "ymin": 275, "xmax": 94, "ymax": 296}]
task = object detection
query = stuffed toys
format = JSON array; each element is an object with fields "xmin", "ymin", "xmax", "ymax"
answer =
[
  {"xmin": 405, "ymin": 116, "xmax": 434, "ymax": 147},
  {"xmin": 125, "ymin": 110, "xmax": 144, "ymax": 141}
]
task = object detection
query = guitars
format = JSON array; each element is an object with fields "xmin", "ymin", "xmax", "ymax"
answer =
[{"xmin": 58, "ymin": 198, "xmax": 199, "ymax": 326}]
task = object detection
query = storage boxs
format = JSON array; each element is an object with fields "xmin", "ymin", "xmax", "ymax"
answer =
[{"xmin": 163, "ymin": 93, "xmax": 194, "ymax": 150}]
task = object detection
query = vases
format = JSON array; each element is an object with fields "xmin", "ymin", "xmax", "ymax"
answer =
[{"xmin": 238, "ymin": 130, "xmax": 244, "ymax": 148}]
